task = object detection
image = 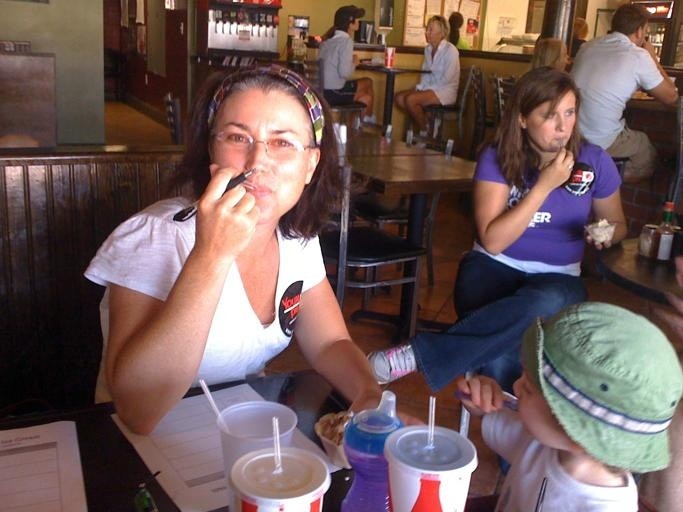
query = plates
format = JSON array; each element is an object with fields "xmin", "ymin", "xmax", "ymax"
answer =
[{"xmin": 631, "ymin": 97, "xmax": 656, "ymax": 102}]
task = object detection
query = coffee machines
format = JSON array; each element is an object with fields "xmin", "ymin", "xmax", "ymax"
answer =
[{"xmin": 354, "ymin": 20, "xmax": 374, "ymax": 43}]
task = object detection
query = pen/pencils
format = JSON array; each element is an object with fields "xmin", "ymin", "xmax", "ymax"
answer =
[{"xmin": 173, "ymin": 169, "xmax": 256, "ymax": 222}]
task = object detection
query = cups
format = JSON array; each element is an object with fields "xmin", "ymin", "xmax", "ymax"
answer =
[
  {"xmin": 640, "ymin": 224, "xmax": 659, "ymax": 256},
  {"xmin": 370, "ymin": 56, "xmax": 383, "ymax": 65},
  {"xmin": 227, "ymin": 447, "xmax": 331, "ymax": 512},
  {"xmin": 583, "ymin": 221, "xmax": 617, "ymax": 242},
  {"xmin": 386, "ymin": 426, "xmax": 479, "ymax": 511},
  {"xmin": 667, "ymin": 78, "xmax": 676, "ymax": 84},
  {"xmin": 384, "ymin": 48, "xmax": 395, "ymax": 68},
  {"xmin": 215, "ymin": 401, "xmax": 300, "ymax": 487}
]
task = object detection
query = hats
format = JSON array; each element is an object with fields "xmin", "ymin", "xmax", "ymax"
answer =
[
  {"xmin": 523, "ymin": 302, "xmax": 682, "ymax": 476},
  {"xmin": 334, "ymin": 5, "xmax": 365, "ymax": 26}
]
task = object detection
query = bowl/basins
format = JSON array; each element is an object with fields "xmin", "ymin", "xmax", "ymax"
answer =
[{"xmin": 312, "ymin": 408, "xmax": 352, "ymax": 469}]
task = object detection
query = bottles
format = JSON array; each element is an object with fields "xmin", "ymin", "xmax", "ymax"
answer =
[
  {"xmin": 338, "ymin": 124, "xmax": 347, "ymax": 145},
  {"xmin": 338, "ymin": 390, "xmax": 405, "ymax": 511},
  {"xmin": 645, "ymin": 25, "xmax": 665, "ymax": 61},
  {"xmin": 652, "ymin": 202, "xmax": 678, "ymax": 264}
]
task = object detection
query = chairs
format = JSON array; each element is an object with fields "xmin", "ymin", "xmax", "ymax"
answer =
[
  {"xmin": 277, "ymin": 60, "xmax": 517, "ymax": 339},
  {"xmin": 0, "ymin": 36, "xmax": 683, "ymax": 511},
  {"xmin": 2, "ymin": 151, "xmax": 206, "ymax": 407}
]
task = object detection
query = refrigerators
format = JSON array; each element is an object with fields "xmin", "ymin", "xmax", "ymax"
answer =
[{"xmin": 630, "ymin": 1, "xmax": 673, "ymax": 68}]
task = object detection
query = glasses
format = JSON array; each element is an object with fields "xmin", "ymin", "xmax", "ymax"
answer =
[{"xmin": 209, "ymin": 128, "xmax": 319, "ymax": 157}]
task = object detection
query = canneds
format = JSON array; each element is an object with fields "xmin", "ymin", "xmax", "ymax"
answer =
[
  {"xmin": 638, "ymin": 224, "xmax": 658, "ymax": 258},
  {"xmin": 670, "ymin": 226, "xmax": 683, "ymax": 256}
]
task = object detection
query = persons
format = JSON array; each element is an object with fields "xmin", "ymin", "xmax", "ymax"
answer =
[
  {"xmin": 367, "ymin": 70, "xmax": 628, "ymax": 391},
  {"xmin": 317, "ymin": 4, "xmax": 378, "ymax": 125},
  {"xmin": 396, "ymin": 16, "xmax": 460, "ymax": 138},
  {"xmin": 84, "ymin": 60, "xmax": 424, "ymax": 447},
  {"xmin": 532, "ymin": 37, "xmax": 571, "ymax": 69},
  {"xmin": 570, "ymin": 17, "xmax": 591, "ymax": 56},
  {"xmin": 457, "ymin": 299, "xmax": 683, "ymax": 512},
  {"xmin": 446, "ymin": 12, "xmax": 472, "ymax": 50},
  {"xmin": 570, "ymin": 4, "xmax": 676, "ymax": 180}
]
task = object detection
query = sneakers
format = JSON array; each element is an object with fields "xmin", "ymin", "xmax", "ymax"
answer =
[{"xmin": 367, "ymin": 343, "xmax": 417, "ymax": 385}]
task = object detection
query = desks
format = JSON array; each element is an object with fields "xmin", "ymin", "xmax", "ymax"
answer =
[
  {"xmin": 0, "ymin": 370, "xmax": 353, "ymax": 511},
  {"xmin": 592, "ymin": 65, "xmax": 683, "ymax": 346}
]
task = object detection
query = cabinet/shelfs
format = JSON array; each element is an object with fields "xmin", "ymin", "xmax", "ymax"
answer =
[{"xmin": 0, "ymin": 41, "xmax": 56, "ymax": 145}]
